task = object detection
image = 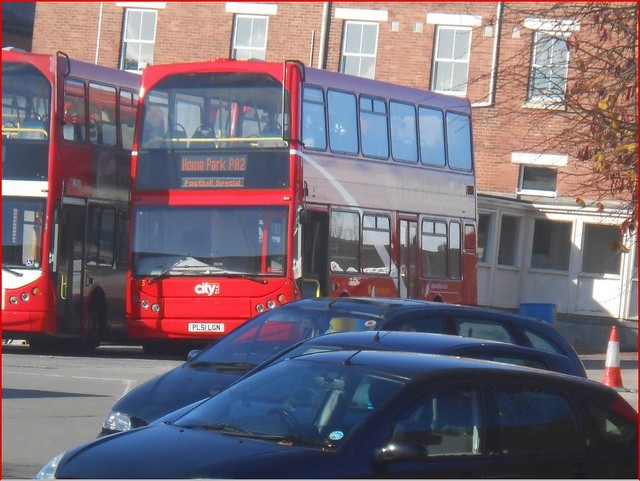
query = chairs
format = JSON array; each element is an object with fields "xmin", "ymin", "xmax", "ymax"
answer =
[
  {"xmin": 428, "ymin": 413, "xmax": 473, "ymax": 456},
  {"xmin": 260, "ymin": 121, "xmax": 282, "ymax": 149},
  {"xmin": 76, "ymin": 116, "xmax": 99, "ymax": 142},
  {"xmin": 161, "ymin": 124, "xmax": 186, "ymax": 149},
  {"xmin": 60, "ymin": 112, "xmax": 76, "ymax": 140},
  {"xmin": 20, "ymin": 113, "xmax": 45, "ymax": 141},
  {"xmin": 190, "ymin": 124, "xmax": 216, "ymax": 148}
]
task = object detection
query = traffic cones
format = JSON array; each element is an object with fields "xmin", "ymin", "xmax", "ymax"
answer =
[{"xmin": 599, "ymin": 325, "xmax": 631, "ymax": 392}]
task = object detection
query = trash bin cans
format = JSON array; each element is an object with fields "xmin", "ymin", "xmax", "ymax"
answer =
[{"xmin": 519, "ymin": 303, "xmax": 556, "ymax": 328}]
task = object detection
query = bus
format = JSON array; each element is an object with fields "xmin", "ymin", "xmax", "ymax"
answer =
[
  {"xmin": 1, "ymin": 46, "xmax": 142, "ymax": 348},
  {"xmin": 126, "ymin": 59, "xmax": 478, "ymax": 354}
]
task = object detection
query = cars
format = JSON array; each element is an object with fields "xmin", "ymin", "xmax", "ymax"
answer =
[{"xmin": 98, "ymin": 256, "xmax": 586, "ymax": 437}]
